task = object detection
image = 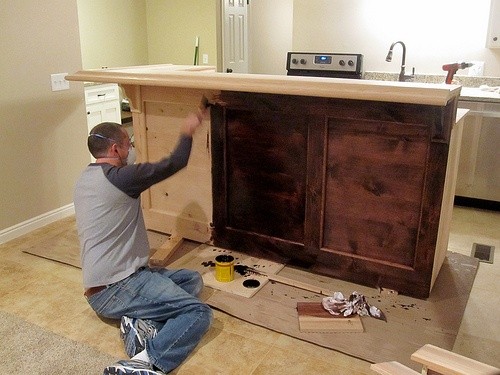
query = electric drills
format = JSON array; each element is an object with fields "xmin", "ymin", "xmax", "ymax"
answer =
[{"xmin": 442, "ymin": 63, "xmax": 476, "ymax": 84}]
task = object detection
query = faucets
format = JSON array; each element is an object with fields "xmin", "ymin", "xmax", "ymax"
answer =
[{"xmin": 385, "ymin": 40, "xmax": 415, "ymax": 82}]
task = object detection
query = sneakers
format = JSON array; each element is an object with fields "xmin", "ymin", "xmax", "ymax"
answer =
[
  {"xmin": 119, "ymin": 315, "xmax": 158, "ymax": 359},
  {"xmin": 103, "ymin": 358, "xmax": 165, "ymax": 375}
]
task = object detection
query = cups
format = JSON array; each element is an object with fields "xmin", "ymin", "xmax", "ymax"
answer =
[{"xmin": 215, "ymin": 254, "xmax": 235, "ymax": 283}]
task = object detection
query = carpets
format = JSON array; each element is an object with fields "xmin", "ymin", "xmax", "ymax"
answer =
[{"xmin": 0, "ymin": 307, "xmax": 120, "ymax": 375}]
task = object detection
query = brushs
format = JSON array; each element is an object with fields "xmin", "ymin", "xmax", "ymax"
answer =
[{"xmin": 197, "ymin": 95, "xmax": 210, "ymax": 119}]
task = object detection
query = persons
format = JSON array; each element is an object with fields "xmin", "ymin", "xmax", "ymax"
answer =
[{"xmin": 73, "ymin": 109, "xmax": 214, "ymax": 375}]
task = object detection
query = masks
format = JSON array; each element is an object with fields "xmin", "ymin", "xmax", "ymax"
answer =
[{"xmin": 116, "ymin": 144, "xmax": 138, "ymax": 164}]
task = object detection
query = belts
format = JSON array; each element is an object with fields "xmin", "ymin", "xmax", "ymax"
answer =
[{"xmin": 83, "ymin": 285, "xmax": 106, "ymax": 298}]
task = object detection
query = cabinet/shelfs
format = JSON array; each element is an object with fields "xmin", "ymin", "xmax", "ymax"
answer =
[{"xmin": 83, "ymin": 83, "xmax": 123, "ymax": 136}]
